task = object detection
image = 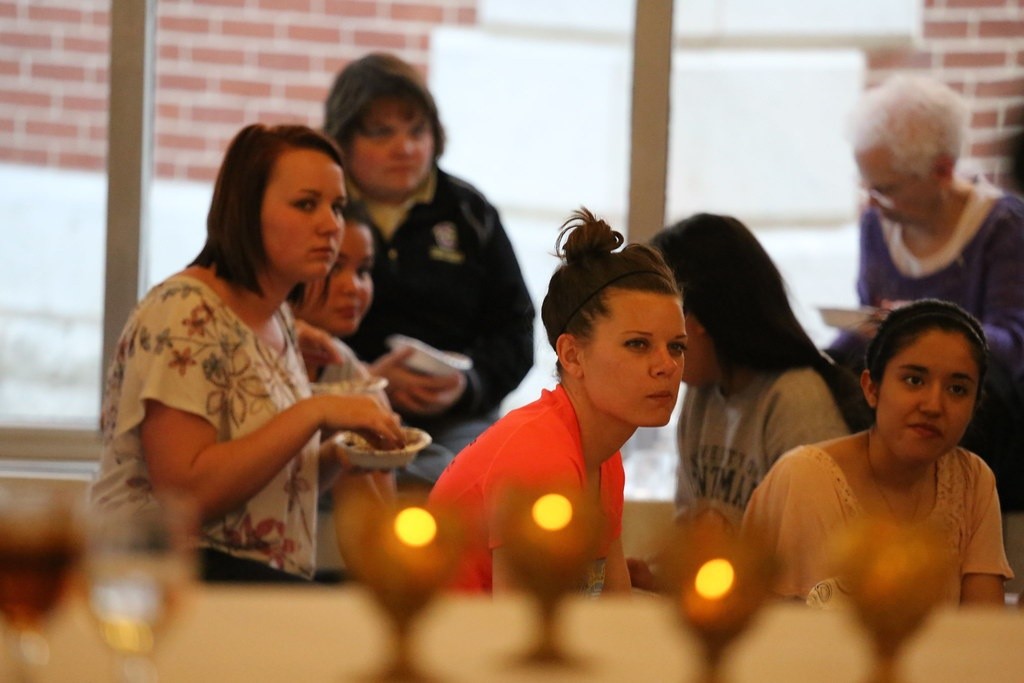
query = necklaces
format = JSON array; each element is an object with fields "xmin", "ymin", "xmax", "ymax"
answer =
[{"xmin": 866, "ymin": 440, "xmax": 927, "ymax": 546}]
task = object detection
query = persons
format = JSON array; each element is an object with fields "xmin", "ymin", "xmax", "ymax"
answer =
[
  {"xmin": 820, "ymin": 82, "xmax": 1024, "ymax": 513},
  {"xmin": 87, "ymin": 54, "xmax": 535, "ymax": 587},
  {"xmin": 415, "ymin": 210, "xmax": 686, "ymax": 664},
  {"xmin": 626, "ymin": 212, "xmax": 865, "ymax": 644},
  {"xmin": 739, "ymin": 302, "xmax": 1015, "ymax": 683}
]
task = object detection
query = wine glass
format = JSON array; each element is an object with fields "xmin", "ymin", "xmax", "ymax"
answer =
[
  {"xmin": 78, "ymin": 510, "xmax": 192, "ymax": 683},
  {"xmin": 0, "ymin": 494, "xmax": 83, "ymax": 683}
]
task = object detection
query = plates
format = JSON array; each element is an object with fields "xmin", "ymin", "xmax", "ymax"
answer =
[
  {"xmin": 334, "ymin": 427, "xmax": 432, "ymax": 468},
  {"xmin": 389, "ymin": 334, "xmax": 473, "ymax": 377},
  {"xmin": 815, "ymin": 306, "xmax": 876, "ymax": 335}
]
